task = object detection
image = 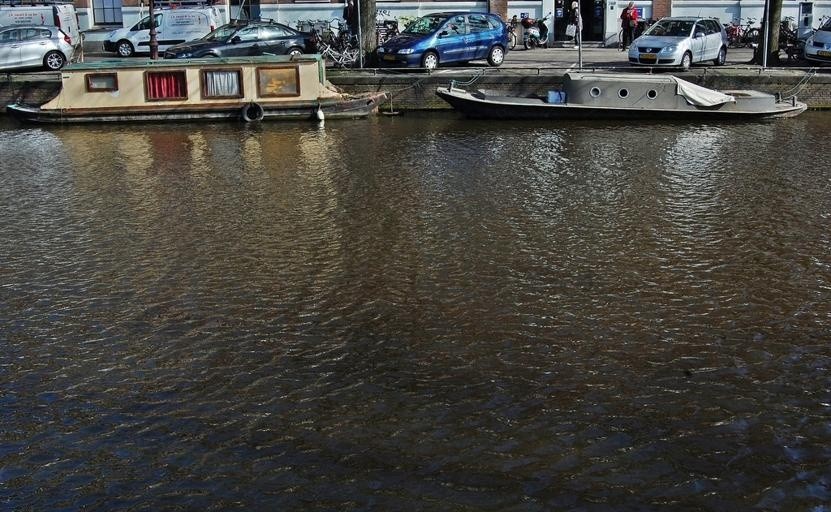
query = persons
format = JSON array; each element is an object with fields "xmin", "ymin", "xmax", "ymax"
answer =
[
  {"xmin": 621, "ymin": 2, "xmax": 639, "ymax": 53},
  {"xmin": 568, "ymin": 1, "xmax": 584, "ymax": 49},
  {"xmin": 343, "ymin": 0, "xmax": 354, "ymax": 35}
]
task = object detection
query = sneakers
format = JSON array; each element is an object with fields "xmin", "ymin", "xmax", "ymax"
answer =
[
  {"xmin": 574, "ymin": 45, "xmax": 579, "ymax": 49},
  {"xmin": 622, "ymin": 49, "xmax": 626, "ymax": 51}
]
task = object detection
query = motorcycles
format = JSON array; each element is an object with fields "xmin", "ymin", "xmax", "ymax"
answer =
[{"xmin": 521, "ymin": 11, "xmax": 553, "ymax": 50}]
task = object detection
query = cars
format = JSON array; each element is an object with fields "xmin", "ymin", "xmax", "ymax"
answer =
[
  {"xmin": 628, "ymin": 17, "xmax": 729, "ymax": 72},
  {"xmin": 374, "ymin": 12, "xmax": 509, "ymax": 72}
]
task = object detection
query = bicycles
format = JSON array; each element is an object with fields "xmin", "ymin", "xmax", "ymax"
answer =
[
  {"xmin": 295, "ymin": 17, "xmax": 370, "ymax": 68},
  {"xmin": 619, "ymin": 23, "xmax": 658, "ymax": 49},
  {"xmin": 724, "ymin": 15, "xmax": 831, "ymax": 64},
  {"xmin": 497, "ymin": 13, "xmax": 517, "ymax": 50}
]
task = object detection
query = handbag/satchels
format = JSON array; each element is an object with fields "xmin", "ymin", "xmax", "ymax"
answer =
[
  {"xmin": 621, "ymin": 16, "xmax": 635, "ymax": 29},
  {"xmin": 565, "ymin": 23, "xmax": 577, "ymax": 38}
]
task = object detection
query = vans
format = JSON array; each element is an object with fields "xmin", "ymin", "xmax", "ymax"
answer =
[{"xmin": 0, "ymin": 2, "xmax": 82, "ymax": 48}]
